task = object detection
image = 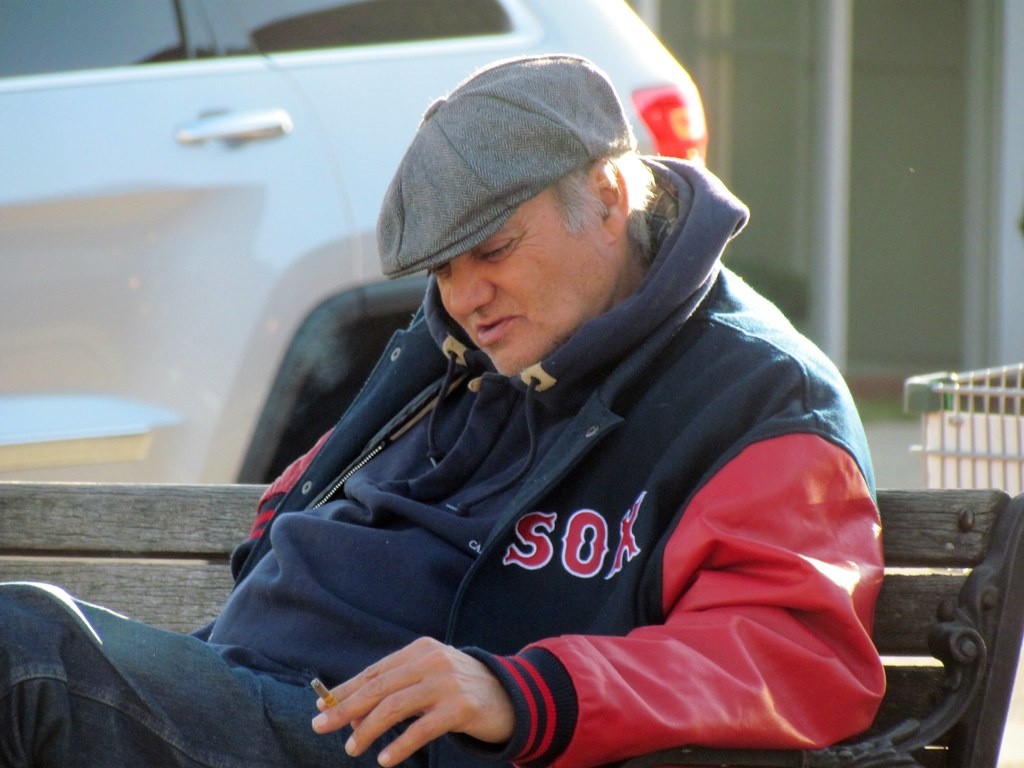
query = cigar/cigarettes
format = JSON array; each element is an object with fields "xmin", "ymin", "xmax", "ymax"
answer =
[{"xmin": 311, "ymin": 676, "xmax": 339, "ymax": 708}]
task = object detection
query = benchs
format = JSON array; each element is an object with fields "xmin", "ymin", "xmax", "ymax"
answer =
[{"xmin": 0, "ymin": 476, "xmax": 1023, "ymax": 768}]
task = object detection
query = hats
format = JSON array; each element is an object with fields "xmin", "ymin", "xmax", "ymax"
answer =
[{"xmin": 376, "ymin": 56, "xmax": 639, "ymax": 278}]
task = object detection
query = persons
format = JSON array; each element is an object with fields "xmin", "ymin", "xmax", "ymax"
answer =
[{"xmin": 0, "ymin": 56, "xmax": 887, "ymax": 768}]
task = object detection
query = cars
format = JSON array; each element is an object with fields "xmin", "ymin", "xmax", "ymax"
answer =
[{"xmin": 0, "ymin": 0, "xmax": 710, "ymax": 491}]
